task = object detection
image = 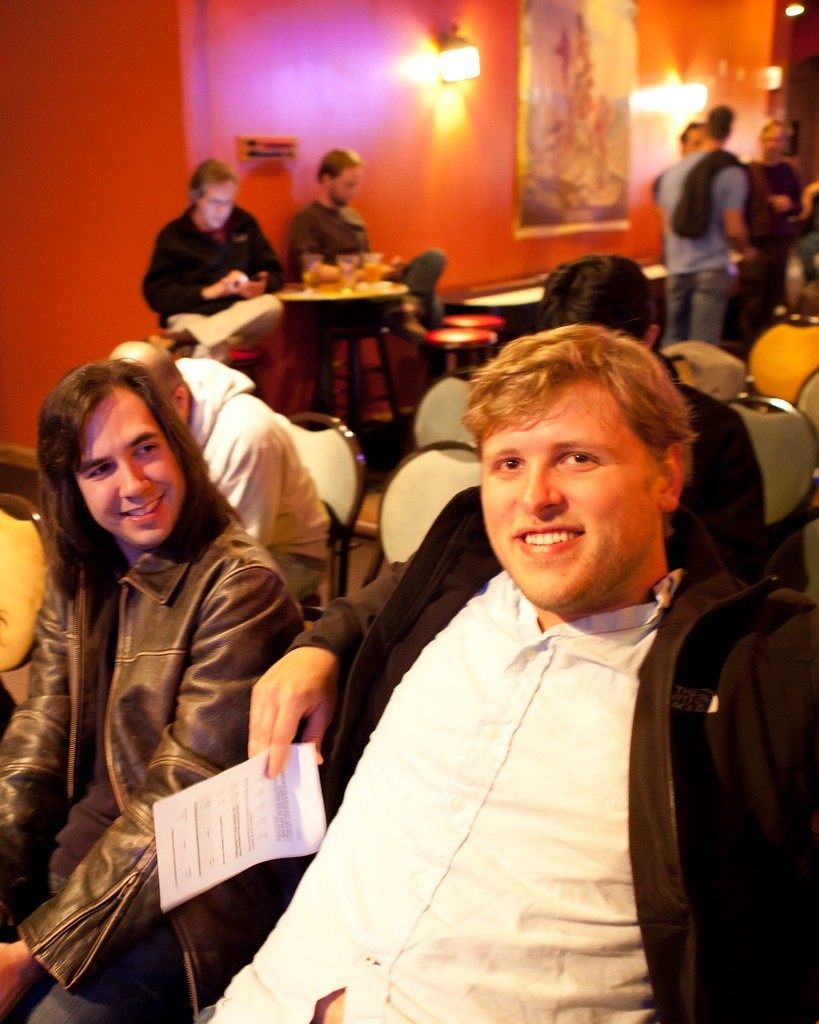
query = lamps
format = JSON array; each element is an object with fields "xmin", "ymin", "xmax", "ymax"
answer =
[{"xmin": 438, "ymin": 28, "xmax": 482, "ymax": 82}]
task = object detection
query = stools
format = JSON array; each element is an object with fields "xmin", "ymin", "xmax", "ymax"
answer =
[
  {"xmin": 424, "ymin": 311, "xmax": 510, "ymax": 378},
  {"xmin": 225, "ymin": 347, "xmax": 270, "ymax": 399}
]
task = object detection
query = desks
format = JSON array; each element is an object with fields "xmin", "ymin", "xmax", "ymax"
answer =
[{"xmin": 287, "ymin": 279, "xmax": 411, "ymax": 463}]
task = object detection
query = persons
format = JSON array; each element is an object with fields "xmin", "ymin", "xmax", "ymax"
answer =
[
  {"xmin": 108, "ymin": 104, "xmax": 819, "ymax": 777},
  {"xmin": 203, "ymin": 322, "xmax": 819, "ymax": 1024},
  {"xmin": 0, "ymin": 352, "xmax": 309, "ymax": 1024}
]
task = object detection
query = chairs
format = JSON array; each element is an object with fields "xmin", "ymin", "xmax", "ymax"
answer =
[
  {"xmin": 414, "ymin": 369, "xmax": 480, "ymax": 448},
  {"xmin": 288, "ymin": 408, "xmax": 367, "ymax": 622},
  {"xmin": 362, "ymin": 442, "xmax": 482, "ymax": 584},
  {"xmin": 0, "ymin": 495, "xmax": 52, "ymax": 678},
  {"xmin": 659, "ymin": 310, "xmax": 818, "ymax": 609}
]
